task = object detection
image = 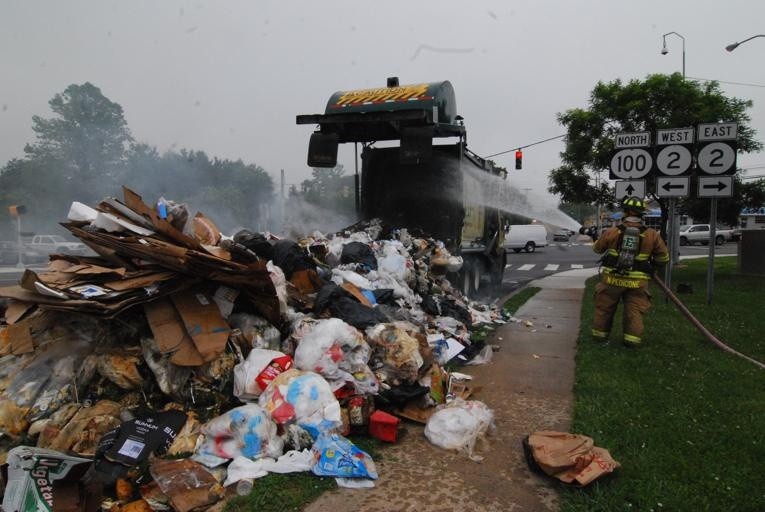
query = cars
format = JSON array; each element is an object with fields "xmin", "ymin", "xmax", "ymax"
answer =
[
  {"xmin": 0, "ymin": 240, "xmax": 42, "ymax": 265},
  {"xmin": 554, "ymin": 229, "xmax": 575, "ymax": 241}
]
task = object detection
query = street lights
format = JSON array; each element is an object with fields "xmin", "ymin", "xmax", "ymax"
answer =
[
  {"xmin": 659, "ymin": 30, "xmax": 685, "ymax": 266},
  {"xmin": 725, "ymin": 34, "xmax": 764, "ymax": 51}
]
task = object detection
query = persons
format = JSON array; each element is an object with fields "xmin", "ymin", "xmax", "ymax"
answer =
[{"xmin": 591, "ymin": 194, "xmax": 669, "ymax": 350}]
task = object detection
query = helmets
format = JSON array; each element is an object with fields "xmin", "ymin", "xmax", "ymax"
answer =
[{"xmin": 621, "ymin": 195, "xmax": 646, "ymax": 214}]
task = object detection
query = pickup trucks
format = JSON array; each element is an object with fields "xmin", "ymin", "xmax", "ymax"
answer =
[
  {"xmin": 23, "ymin": 234, "xmax": 87, "ymax": 253},
  {"xmin": 680, "ymin": 224, "xmax": 734, "ymax": 245}
]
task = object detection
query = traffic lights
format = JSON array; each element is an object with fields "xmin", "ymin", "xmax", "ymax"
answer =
[
  {"xmin": 9, "ymin": 205, "xmax": 26, "ymax": 217},
  {"xmin": 516, "ymin": 151, "xmax": 522, "ymax": 169}
]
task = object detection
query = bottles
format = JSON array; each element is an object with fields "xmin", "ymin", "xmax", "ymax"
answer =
[{"xmin": 236, "ymin": 479, "xmax": 255, "ymax": 497}]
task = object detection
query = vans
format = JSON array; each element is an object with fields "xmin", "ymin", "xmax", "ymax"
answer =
[{"xmin": 504, "ymin": 224, "xmax": 548, "ymax": 252}]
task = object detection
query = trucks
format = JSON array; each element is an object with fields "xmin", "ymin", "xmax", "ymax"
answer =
[{"xmin": 296, "ymin": 77, "xmax": 508, "ymax": 297}]
